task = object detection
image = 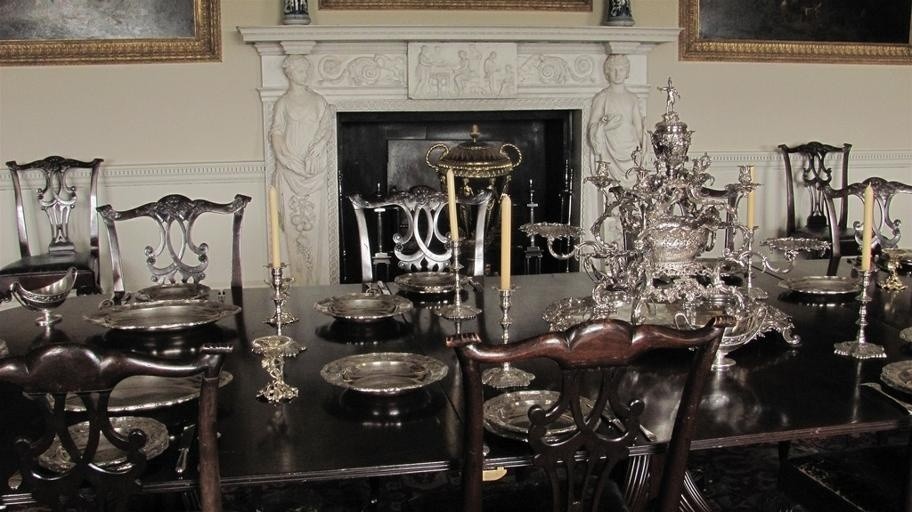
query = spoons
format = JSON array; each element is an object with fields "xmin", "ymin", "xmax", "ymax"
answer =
[{"xmin": 629, "ymin": 399, "xmax": 645, "ymax": 416}]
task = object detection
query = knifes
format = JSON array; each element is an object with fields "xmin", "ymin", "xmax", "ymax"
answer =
[{"xmin": 175, "ymin": 423, "xmax": 195, "ymax": 473}]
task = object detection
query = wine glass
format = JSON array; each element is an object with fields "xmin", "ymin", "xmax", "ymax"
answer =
[{"xmin": 9, "ymin": 266, "xmax": 78, "ymax": 326}]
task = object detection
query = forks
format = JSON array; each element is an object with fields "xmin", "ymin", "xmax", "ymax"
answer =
[{"xmin": 857, "ymin": 382, "xmax": 912, "ymax": 415}]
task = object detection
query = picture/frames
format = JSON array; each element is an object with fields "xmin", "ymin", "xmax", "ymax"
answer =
[
  {"xmin": 678, "ymin": 1, "xmax": 911, "ymax": 66},
  {"xmin": 0, "ymin": 0, "xmax": 223, "ymax": 68}
]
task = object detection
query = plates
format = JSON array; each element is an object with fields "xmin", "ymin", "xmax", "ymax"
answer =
[
  {"xmin": 880, "ymin": 360, "xmax": 912, "ymax": 390},
  {"xmin": 82, "ymin": 283, "xmax": 243, "ymax": 333},
  {"xmin": 23, "ymin": 369, "xmax": 235, "ymax": 415},
  {"xmin": 35, "ymin": 416, "xmax": 170, "ymax": 475},
  {"xmin": 320, "ymin": 352, "xmax": 449, "ymax": 394},
  {"xmin": 482, "ymin": 390, "xmax": 601, "ymax": 443},
  {"xmin": 394, "ymin": 272, "xmax": 470, "ymax": 293},
  {"xmin": 777, "ymin": 275, "xmax": 862, "ymax": 295},
  {"xmin": 313, "ymin": 293, "xmax": 414, "ymax": 322}
]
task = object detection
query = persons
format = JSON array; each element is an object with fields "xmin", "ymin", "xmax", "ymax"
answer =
[
  {"xmin": 413, "ymin": 45, "xmax": 514, "ymax": 96},
  {"xmin": 267, "ymin": 54, "xmax": 334, "ymax": 285},
  {"xmin": 584, "ymin": 52, "xmax": 646, "ymax": 268}
]
